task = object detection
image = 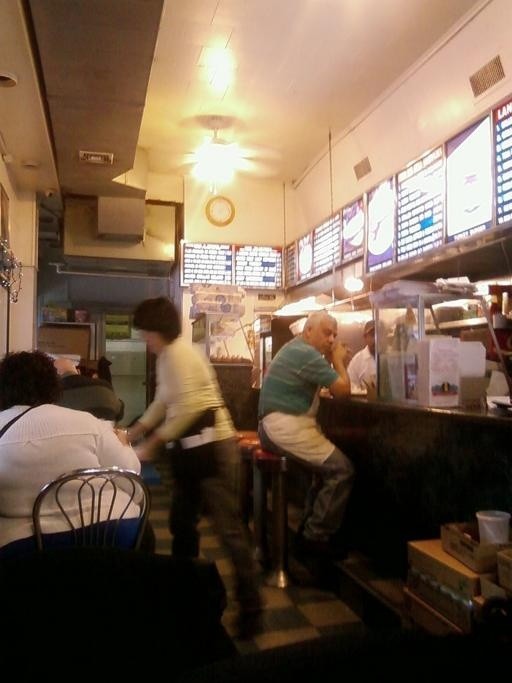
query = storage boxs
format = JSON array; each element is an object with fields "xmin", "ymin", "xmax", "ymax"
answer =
[
  {"xmin": 440, "ymin": 523, "xmax": 512, "ymax": 572},
  {"xmin": 497, "ymin": 547, "xmax": 512, "ymax": 590},
  {"xmin": 406, "ymin": 521, "xmax": 480, "ymax": 599},
  {"xmin": 480, "ymin": 572, "xmax": 512, "ymax": 600},
  {"xmin": 472, "ymin": 595, "xmax": 486, "ymax": 619}
]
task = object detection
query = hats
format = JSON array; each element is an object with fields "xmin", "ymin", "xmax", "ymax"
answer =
[
  {"xmin": 364, "ymin": 321, "xmax": 392, "ymax": 335},
  {"xmin": 75, "ymin": 359, "xmax": 99, "ymax": 372}
]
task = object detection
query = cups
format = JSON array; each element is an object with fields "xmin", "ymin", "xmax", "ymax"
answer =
[
  {"xmin": 459, "ymin": 367, "xmax": 491, "ymax": 414},
  {"xmin": 477, "ymin": 512, "xmax": 510, "ymax": 545}
]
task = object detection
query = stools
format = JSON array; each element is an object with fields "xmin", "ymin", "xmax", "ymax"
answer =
[
  {"xmin": 238, "ymin": 438, "xmax": 273, "ymax": 573},
  {"xmin": 253, "ymin": 450, "xmax": 308, "ymax": 589},
  {"xmin": 236, "ymin": 428, "xmax": 258, "ymax": 439}
]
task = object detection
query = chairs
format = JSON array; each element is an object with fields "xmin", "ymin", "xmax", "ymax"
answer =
[{"xmin": 31, "ymin": 467, "xmax": 151, "ymax": 563}]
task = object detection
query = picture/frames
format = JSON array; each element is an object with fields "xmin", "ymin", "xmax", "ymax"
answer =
[
  {"xmin": 395, "ymin": 133, "xmax": 446, "ymax": 262},
  {"xmin": 490, "ymin": 91, "xmax": 512, "ymax": 232},
  {"xmin": 297, "ymin": 227, "xmax": 313, "ymax": 285},
  {"xmin": 179, "ymin": 239, "xmax": 233, "ymax": 288},
  {"xmin": 312, "ymin": 207, "xmax": 342, "ymax": 279},
  {"xmin": 364, "ymin": 169, "xmax": 398, "ymax": 278},
  {"xmin": 284, "ymin": 239, "xmax": 297, "ymax": 289},
  {"xmin": 342, "ymin": 191, "xmax": 364, "ymax": 265},
  {"xmin": 233, "ymin": 243, "xmax": 284, "ymax": 291},
  {"xmin": 442, "ymin": 99, "xmax": 497, "ymax": 246}
]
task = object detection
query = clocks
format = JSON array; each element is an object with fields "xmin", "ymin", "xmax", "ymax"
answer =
[{"xmin": 206, "ymin": 195, "xmax": 236, "ymax": 228}]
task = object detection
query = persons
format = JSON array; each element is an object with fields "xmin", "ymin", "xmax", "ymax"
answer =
[
  {"xmin": 344, "ymin": 319, "xmax": 388, "ymax": 391},
  {"xmin": 114, "ymin": 294, "xmax": 239, "ymax": 558},
  {"xmin": 51, "ymin": 357, "xmax": 126, "ymax": 422},
  {"xmin": 0, "ymin": 349, "xmax": 147, "ymax": 560},
  {"xmin": 255, "ymin": 308, "xmax": 359, "ymax": 571}
]
